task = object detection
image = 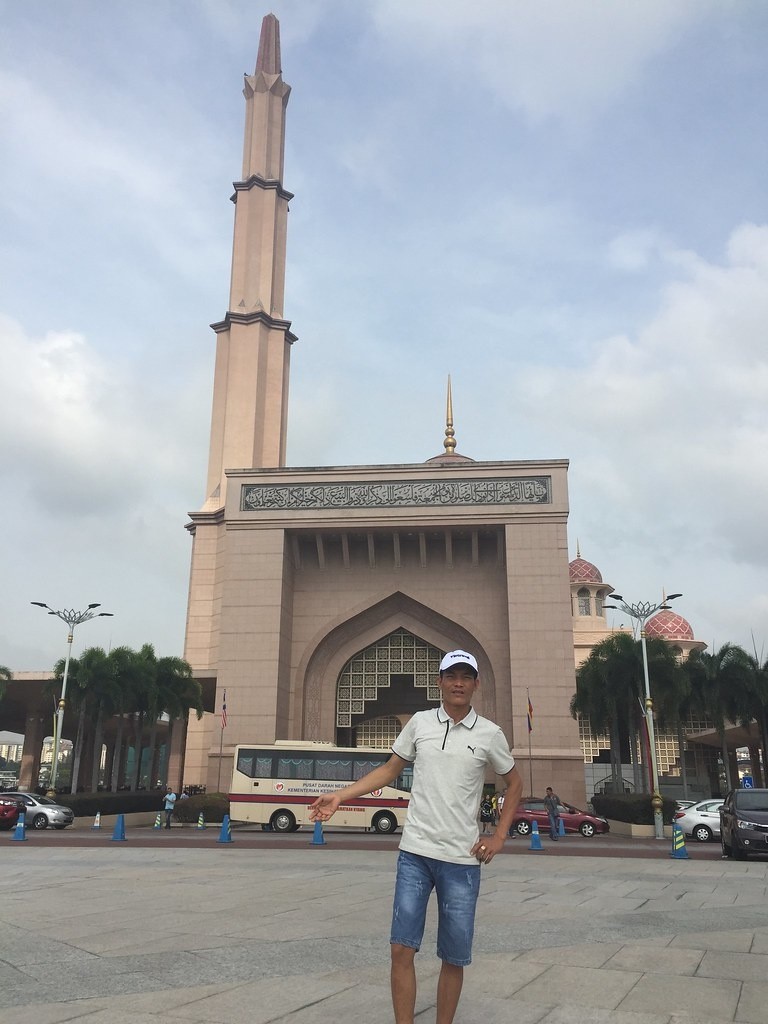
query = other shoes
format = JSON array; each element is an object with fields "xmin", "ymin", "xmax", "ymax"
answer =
[{"xmin": 510, "ymin": 835, "xmax": 516, "ymax": 838}]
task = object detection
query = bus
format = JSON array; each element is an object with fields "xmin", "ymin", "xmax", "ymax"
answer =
[{"xmin": 228, "ymin": 739, "xmax": 416, "ymax": 833}]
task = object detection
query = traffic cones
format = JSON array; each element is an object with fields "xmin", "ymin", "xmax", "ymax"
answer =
[
  {"xmin": 90, "ymin": 811, "xmax": 103, "ymax": 830},
  {"xmin": 216, "ymin": 815, "xmax": 235, "ymax": 843},
  {"xmin": 110, "ymin": 814, "xmax": 129, "ymax": 841},
  {"xmin": 196, "ymin": 812, "xmax": 207, "ymax": 830},
  {"xmin": 668, "ymin": 825, "xmax": 690, "ymax": 859},
  {"xmin": 309, "ymin": 821, "xmax": 326, "ymax": 846},
  {"xmin": 8, "ymin": 813, "xmax": 28, "ymax": 841},
  {"xmin": 527, "ymin": 821, "xmax": 546, "ymax": 851},
  {"xmin": 151, "ymin": 812, "xmax": 163, "ymax": 830}
]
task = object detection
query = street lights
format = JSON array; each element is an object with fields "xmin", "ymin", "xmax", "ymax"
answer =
[
  {"xmin": 600, "ymin": 592, "xmax": 684, "ymax": 840},
  {"xmin": 30, "ymin": 600, "xmax": 115, "ymax": 799}
]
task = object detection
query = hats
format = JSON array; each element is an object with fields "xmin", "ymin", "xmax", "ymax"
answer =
[{"xmin": 439, "ymin": 649, "xmax": 478, "ymax": 677}]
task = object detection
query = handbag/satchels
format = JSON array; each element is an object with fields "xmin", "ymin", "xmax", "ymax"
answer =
[{"xmin": 554, "ymin": 808, "xmax": 559, "ymax": 817}]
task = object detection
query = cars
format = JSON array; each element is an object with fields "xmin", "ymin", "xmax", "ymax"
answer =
[
  {"xmin": 0, "ymin": 792, "xmax": 75, "ymax": 830},
  {"xmin": 0, "ymin": 796, "xmax": 28, "ymax": 830},
  {"xmin": 511, "ymin": 798, "xmax": 611, "ymax": 838},
  {"xmin": 671, "ymin": 799, "xmax": 726, "ymax": 842},
  {"xmin": 718, "ymin": 788, "xmax": 768, "ymax": 861}
]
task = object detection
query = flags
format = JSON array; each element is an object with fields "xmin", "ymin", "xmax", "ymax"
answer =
[
  {"xmin": 221, "ymin": 691, "xmax": 227, "ymax": 729},
  {"xmin": 527, "ymin": 695, "xmax": 534, "ymax": 734}
]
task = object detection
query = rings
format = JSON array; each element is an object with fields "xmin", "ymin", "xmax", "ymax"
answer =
[{"xmin": 481, "ymin": 846, "xmax": 486, "ymax": 850}]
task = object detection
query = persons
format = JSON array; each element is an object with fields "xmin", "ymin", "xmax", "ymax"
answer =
[
  {"xmin": 309, "ymin": 650, "xmax": 523, "ymax": 1024},
  {"xmin": 162, "ymin": 787, "xmax": 176, "ymax": 829},
  {"xmin": 543, "ymin": 787, "xmax": 567, "ymax": 841},
  {"xmin": 180, "ymin": 791, "xmax": 190, "ymax": 800},
  {"xmin": 492, "ymin": 788, "xmax": 516, "ymax": 839},
  {"xmin": 478, "ymin": 794, "xmax": 494, "ymax": 834}
]
